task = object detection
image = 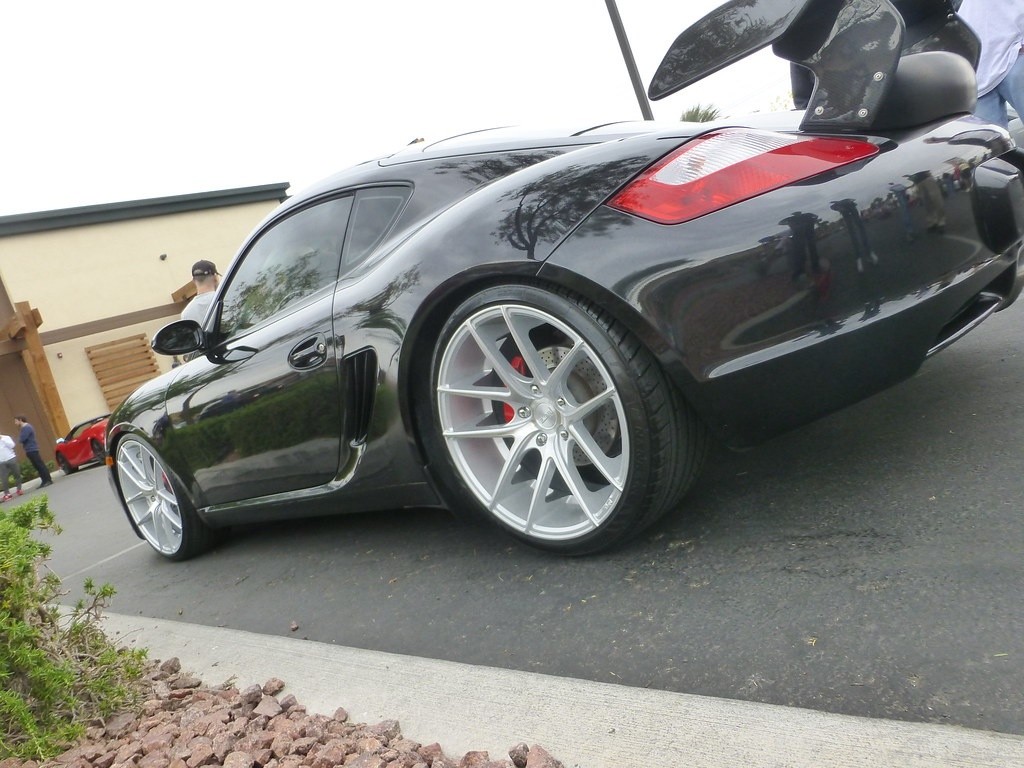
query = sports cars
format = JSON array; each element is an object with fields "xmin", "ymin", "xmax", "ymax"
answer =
[
  {"xmin": 102, "ymin": 0, "xmax": 1024, "ymax": 561},
  {"xmin": 55, "ymin": 413, "xmax": 110, "ymax": 475}
]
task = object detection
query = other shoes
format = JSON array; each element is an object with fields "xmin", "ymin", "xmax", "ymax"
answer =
[
  {"xmin": 16, "ymin": 490, "xmax": 24, "ymax": 495},
  {"xmin": 3, "ymin": 493, "xmax": 12, "ymax": 501},
  {"xmin": 36, "ymin": 484, "xmax": 46, "ymax": 489},
  {"xmin": 44, "ymin": 480, "xmax": 53, "ymax": 487}
]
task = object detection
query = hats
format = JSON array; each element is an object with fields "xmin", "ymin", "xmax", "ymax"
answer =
[{"xmin": 193, "ymin": 260, "xmax": 222, "ymax": 276}]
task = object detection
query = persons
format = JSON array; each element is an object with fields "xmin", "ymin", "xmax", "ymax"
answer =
[
  {"xmin": 172, "ymin": 355, "xmax": 182, "ymax": 369},
  {"xmin": 0, "ymin": 434, "xmax": 24, "ymax": 503},
  {"xmin": 14, "ymin": 415, "xmax": 53, "ymax": 490},
  {"xmin": 953, "ymin": 0, "xmax": 1024, "ymax": 137},
  {"xmin": 179, "ymin": 259, "xmax": 223, "ymax": 329}
]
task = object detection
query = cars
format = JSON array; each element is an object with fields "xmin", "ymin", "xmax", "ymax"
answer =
[{"xmin": 169, "ymin": 381, "xmax": 287, "ymax": 430}]
